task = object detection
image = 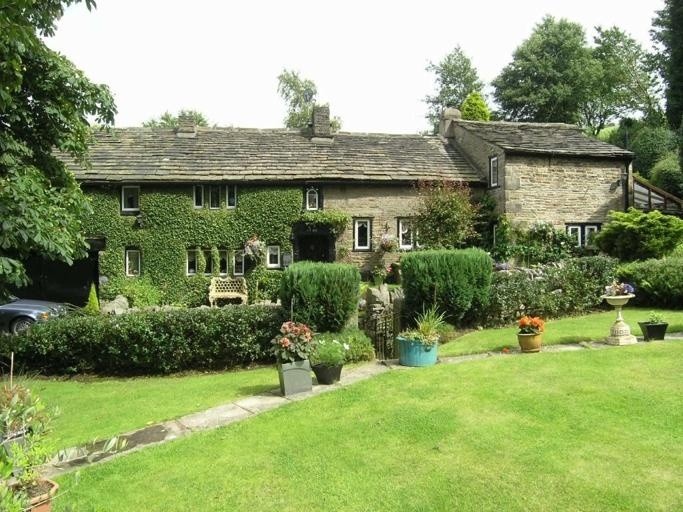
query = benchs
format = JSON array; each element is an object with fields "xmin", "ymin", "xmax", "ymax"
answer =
[{"xmin": 208, "ymin": 277, "xmax": 249, "ymax": 307}]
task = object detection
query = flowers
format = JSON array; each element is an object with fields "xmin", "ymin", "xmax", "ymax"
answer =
[
  {"xmin": 272, "ymin": 320, "xmax": 313, "ymax": 364},
  {"xmin": 517, "ymin": 314, "xmax": 545, "ymax": 334},
  {"xmin": 606, "ymin": 280, "xmax": 634, "ymax": 295}
]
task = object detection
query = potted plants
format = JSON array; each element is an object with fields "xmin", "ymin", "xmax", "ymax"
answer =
[
  {"xmin": 0, "ymin": 385, "xmax": 45, "ymax": 464},
  {"xmin": 395, "ymin": 302, "xmax": 450, "ymax": 366},
  {"xmin": 637, "ymin": 311, "xmax": 669, "ymax": 341},
  {"xmin": 0, "ymin": 455, "xmax": 58, "ymax": 512},
  {"xmin": 307, "ymin": 331, "xmax": 351, "ymax": 385}
]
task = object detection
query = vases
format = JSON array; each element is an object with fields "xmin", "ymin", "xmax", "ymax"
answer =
[
  {"xmin": 517, "ymin": 334, "xmax": 543, "ymax": 353},
  {"xmin": 601, "ymin": 295, "xmax": 638, "ymax": 346},
  {"xmin": 277, "ymin": 358, "xmax": 313, "ymax": 396}
]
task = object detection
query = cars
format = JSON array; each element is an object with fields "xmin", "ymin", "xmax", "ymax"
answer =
[{"xmin": 0, "ymin": 287, "xmax": 69, "ymax": 340}]
